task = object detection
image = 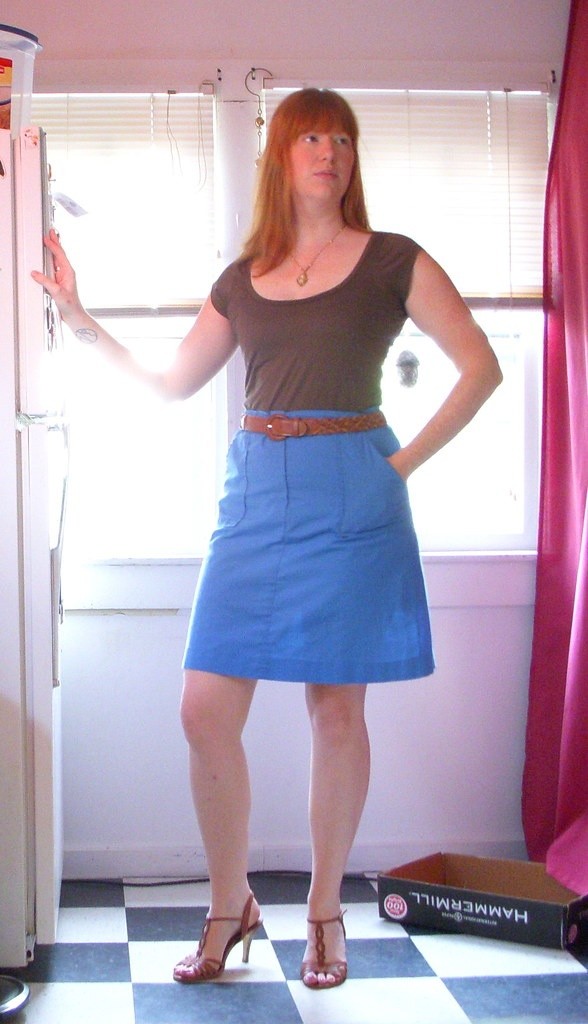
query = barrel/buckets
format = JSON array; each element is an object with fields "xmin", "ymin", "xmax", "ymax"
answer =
[{"xmin": 0, "ymin": 23, "xmax": 42, "ymax": 132}]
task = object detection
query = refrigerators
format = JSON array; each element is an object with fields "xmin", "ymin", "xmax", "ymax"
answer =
[{"xmin": 2, "ymin": 126, "xmax": 64, "ymax": 970}]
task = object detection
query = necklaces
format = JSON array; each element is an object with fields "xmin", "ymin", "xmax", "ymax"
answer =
[{"xmin": 287, "ymin": 226, "xmax": 346, "ymax": 286}]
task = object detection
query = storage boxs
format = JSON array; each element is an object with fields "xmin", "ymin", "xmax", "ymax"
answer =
[{"xmin": 377, "ymin": 851, "xmax": 588, "ymax": 949}]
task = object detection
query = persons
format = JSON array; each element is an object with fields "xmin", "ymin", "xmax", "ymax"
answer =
[{"xmin": 29, "ymin": 88, "xmax": 504, "ymax": 991}]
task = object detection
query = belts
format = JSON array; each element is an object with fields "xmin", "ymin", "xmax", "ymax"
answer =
[{"xmin": 240, "ymin": 411, "xmax": 388, "ymax": 437}]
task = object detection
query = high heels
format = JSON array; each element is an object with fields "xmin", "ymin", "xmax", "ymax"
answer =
[
  {"xmin": 174, "ymin": 891, "xmax": 262, "ymax": 981},
  {"xmin": 300, "ymin": 911, "xmax": 347, "ymax": 990}
]
